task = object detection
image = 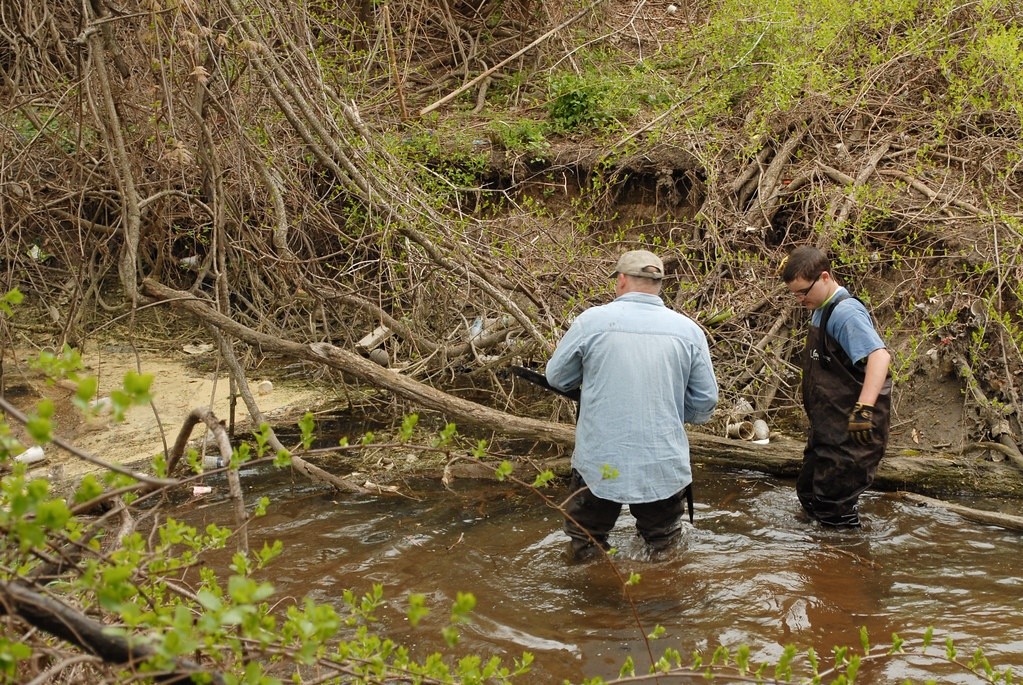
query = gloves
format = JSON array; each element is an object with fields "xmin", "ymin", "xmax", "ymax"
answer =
[{"xmin": 848, "ymin": 402, "xmax": 883, "ymax": 448}]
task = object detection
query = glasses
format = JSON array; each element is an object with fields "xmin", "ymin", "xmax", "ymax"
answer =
[{"xmin": 789, "ymin": 273, "xmax": 822, "ymax": 298}]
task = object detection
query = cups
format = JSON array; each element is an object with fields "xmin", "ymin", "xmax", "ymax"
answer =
[
  {"xmin": 755, "ymin": 420, "xmax": 770, "ymax": 439},
  {"xmin": 729, "ymin": 421, "xmax": 754, "ymax": 440},
  {"xmin": 735, "ymin": 397, "xmax": 754, "ymax": 418},
  {"xmin": 16, "ymin": 444, "xmax": 44, "ymax": 465},
  {"xmin": 971, "ymin": 298, "xmax": 987, "ymax": 317}
]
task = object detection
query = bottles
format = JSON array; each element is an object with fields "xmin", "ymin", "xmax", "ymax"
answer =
[
  {"xmin": 89, "ymin": 396, "xmax": 113, "ymax": 411},
  {"xmin": 201, "ymin": 456, "xmax": 224, "ymax": 470}
]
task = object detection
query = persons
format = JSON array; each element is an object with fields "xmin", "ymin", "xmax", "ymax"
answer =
[
  {"xmin": 781, "ymin": 246, "xmax": 894, "ymax": 532},
  {"xmin": 545, "ymin": 249, "xmax": 719, "ymax": 564}
]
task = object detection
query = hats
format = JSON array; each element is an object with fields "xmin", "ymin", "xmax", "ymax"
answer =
[{"xmin": 607, "ymin": 250, "xmax": 664, "ymax": 279}]
